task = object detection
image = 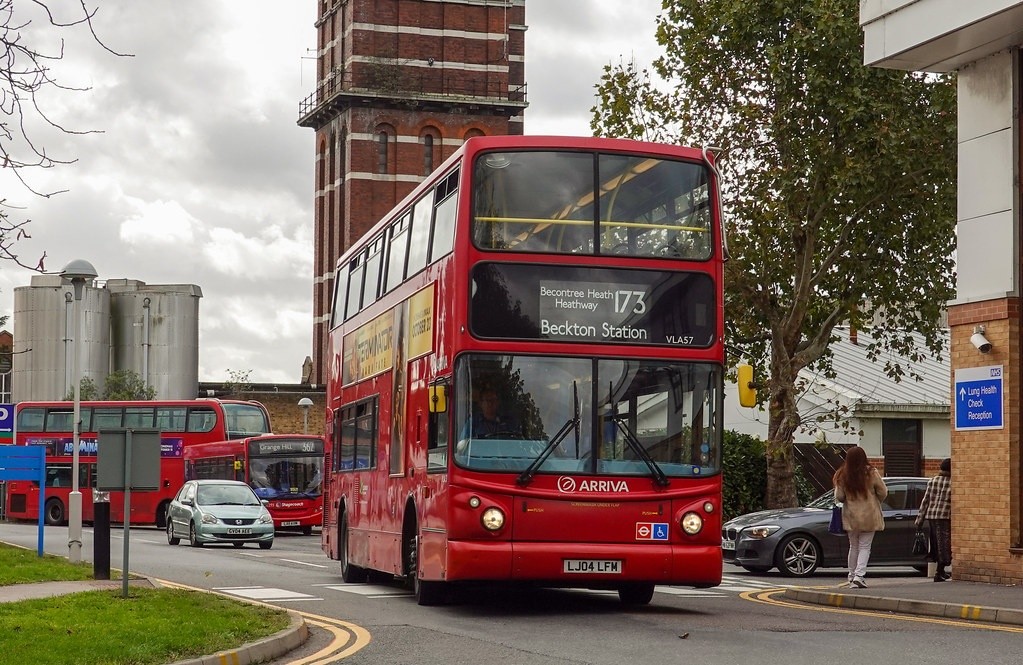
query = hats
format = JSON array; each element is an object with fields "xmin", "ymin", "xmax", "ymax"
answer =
[{"xmin": 940, "ymin": 458, "xmax": 951, "ymax": 471}]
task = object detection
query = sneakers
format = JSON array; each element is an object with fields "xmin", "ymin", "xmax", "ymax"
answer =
[
  {"xmin": 849, "ymin": 579, "xmax": 859, "ymax": 589},
  {"xmin": 852, "ymin": 575, "xmax": 869, "ymax": 588}
]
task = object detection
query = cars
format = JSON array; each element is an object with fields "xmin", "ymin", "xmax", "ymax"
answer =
[
  {"xmin": 166, "ymin": 479, "xmax": 275, "ymax": 549},
  {"xmin": 722, "ymin": 476, "xmax": 935, "ymax": 579}
]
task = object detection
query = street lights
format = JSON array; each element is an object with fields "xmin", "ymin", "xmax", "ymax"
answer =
[
  {"xmin": 297, "ymin": 397, "xmax": 314, "ymax": 435},
  {"xmin": 58, "ymin": 259, "xmax": 99, "ymax": 562}
]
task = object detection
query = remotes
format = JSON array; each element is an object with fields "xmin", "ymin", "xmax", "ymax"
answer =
[{"xmin": 970, "ymin": 333, "xmax": 992, "ymax": 355}]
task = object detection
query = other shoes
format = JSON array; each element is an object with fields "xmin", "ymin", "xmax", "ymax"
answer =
[{"xmin": 934, "ymin": 571, "xmax": 951, "ymax": 582}]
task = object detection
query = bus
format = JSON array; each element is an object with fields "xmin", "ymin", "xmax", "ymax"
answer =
[
  {"xmin": 4, "ymin": 397, "xmax": 273, "ymax": 528},
  {"xmin": 321, "ymin": 135, "xmax": 760, "ymax": 607},
  {"xmin": 182, "ymin": 431, "xmax": 325, "ymax": 535}
]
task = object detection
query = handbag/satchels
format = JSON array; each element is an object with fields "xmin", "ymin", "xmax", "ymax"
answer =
[
  {"xmin": 911, "ymin": 527, "xmax": 929, "ymax": 555},
  {"xmin": 827, "ymin": 502, "xmax": 847, "ymax": 536}
]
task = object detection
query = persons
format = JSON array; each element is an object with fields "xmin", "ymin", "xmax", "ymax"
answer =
[
  {"xmin": 912, "ymin": 458, "xmax": 952, "ymax": 583},
  {"xmin": 459, "ymin": 386, "xmax": 517, "ymax": 442},
  {"xmin": 274, "ymin": 474, "xmax": 285, "ymax": 490},
  {"xmin": 833, "ymin": 446, "xmax": 887, "ymax": 588}
]
